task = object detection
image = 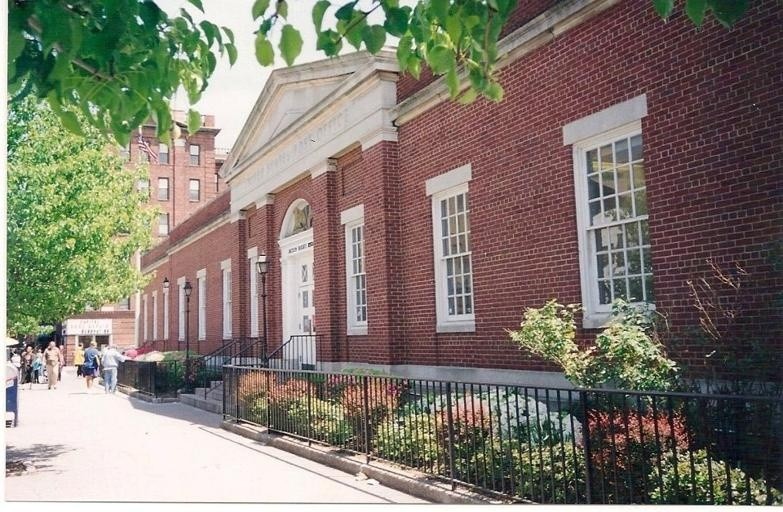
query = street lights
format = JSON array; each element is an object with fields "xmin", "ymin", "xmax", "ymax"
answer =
[
  {"xmin": 256, "ymin": 254, "xmax": 270, "ymax": 376},
  {"xmin": 183, "ymin": 281, "xmax": 192, "ymax": 394}
]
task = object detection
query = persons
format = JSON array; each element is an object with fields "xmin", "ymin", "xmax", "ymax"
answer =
[
  {"xmin": 292, "ymin": 208, "xmax": 308, "ymax": 233},
  {"xmin": 81, "ymin": 340, "xmax": 102, "ymax": 389},
  {"xmin": 8, "ymin": 340, "xmax": 66, "ymax": 389},
  {"xmin": 100, "ymin": 344, "xmax": 125, "ymax": 394},
  {"xmin": 120, "ymin": 351, "xmax": 131, "ymax": 362},
  {"xmin": 73, "ymin": 342, "xmax": 85, "ymax": 377}
]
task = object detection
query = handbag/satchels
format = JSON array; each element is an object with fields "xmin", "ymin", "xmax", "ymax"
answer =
[{"xmin": 94, "ymin": 354, "xmax": 101, "ymax": 367}]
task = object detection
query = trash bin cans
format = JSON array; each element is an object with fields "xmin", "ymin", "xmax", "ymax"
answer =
[{"xmin": 5, "ymin": 363, "xmax": 18, "ymax": 428}]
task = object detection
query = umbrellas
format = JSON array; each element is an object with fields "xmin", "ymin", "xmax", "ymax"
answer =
[{"xmin": 5, "ymin": 337, "xmax": 19, "ymax": 346}]
